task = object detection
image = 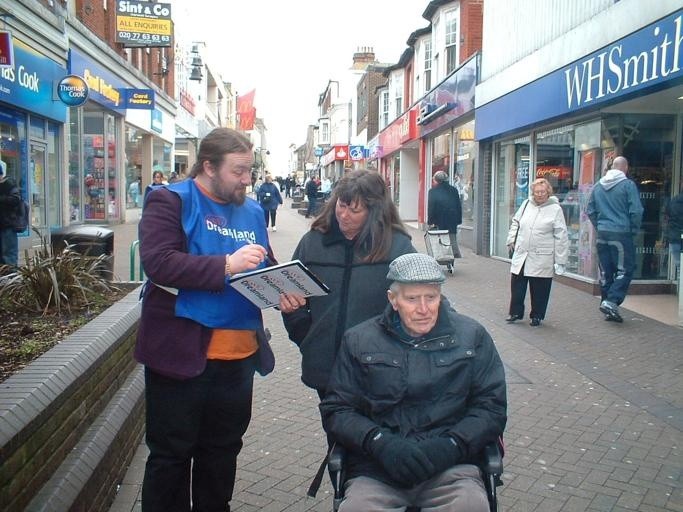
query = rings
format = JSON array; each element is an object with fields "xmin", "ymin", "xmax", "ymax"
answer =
[{"xmin": 292, "ymin": 306, "xmax": 299, "ymax": 309}]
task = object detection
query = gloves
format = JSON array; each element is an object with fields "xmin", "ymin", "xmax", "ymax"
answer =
[
  {"xmin": 367, "ymin": 427, "xmax": 435, "ymax": 489},
  {"xmin": 416, "ymin": 436, "xmax": 462, "ymax": 479}
]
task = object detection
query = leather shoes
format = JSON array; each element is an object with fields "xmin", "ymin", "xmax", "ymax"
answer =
[
  {"xmin": 506, "ymin": 313, "xmax": 523, "ymax": 321},
  {"xmin": 530, "ymin": 318, "xmax": 542, "ymax": 327}
]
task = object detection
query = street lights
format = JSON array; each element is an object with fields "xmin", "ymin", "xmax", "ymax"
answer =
[{"xmin": 253, "ymin": 147, "xmax": 270, "ymax": 164}]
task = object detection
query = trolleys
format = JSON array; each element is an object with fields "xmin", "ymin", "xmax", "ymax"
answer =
[{"xmin": 424, "ymin": 223, "xmax": 454, "ymax": 276}]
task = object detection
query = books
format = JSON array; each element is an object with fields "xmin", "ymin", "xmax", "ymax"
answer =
[{"xmin": 227, "ymin": 259, "xmax": 329, "ymax": 309}]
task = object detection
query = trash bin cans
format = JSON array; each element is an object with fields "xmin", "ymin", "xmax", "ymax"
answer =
[{"xmin": 50, "ymin": 225, "xmax": 114, "ymax": 280}]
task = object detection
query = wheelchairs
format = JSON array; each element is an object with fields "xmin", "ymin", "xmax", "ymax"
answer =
[{"xmin": 325, "ymin": 309, "xmax": 505, "ymax": 512}]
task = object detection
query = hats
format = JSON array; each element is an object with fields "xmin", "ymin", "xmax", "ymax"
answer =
[
  {"xmin": 387, "ymin": 253, "xmax": 445, "ymax": 285},
  {"xmin": 436, "ymin": 171, "xmax": 448, "ymax": 180}
]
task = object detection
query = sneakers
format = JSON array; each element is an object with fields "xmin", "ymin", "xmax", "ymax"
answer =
[
  {"xmin": 272, "ymin": 226, "xmax": 276, "ymax": 231},
  {"xmin": 600, "ymin": 301, "xmax": 623, "ymax": 323}
]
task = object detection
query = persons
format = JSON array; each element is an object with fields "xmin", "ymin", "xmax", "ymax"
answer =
[
  {"xmin": 427, "ymin": 170, "xmax": 462, "ymax": 273},
  {"xmin": 665, "ymin": 192, "xmax": 683, "ymax": 294},
  {"xmin": 134, "ymin": 127, "xmax": 306, "ymax": 512},
  {"xmin": 253, "ymin": 174, "xmax": 341, "ymax": 232},
  {"xmin": 0, "ymin": 159, "xmax": 21, "ymax": 269},
  {"xmin": 505, "ymin": 178, "xmax": 569, "ymax": 326},
  {"xmin": 319, "ymin": 253, "xmax": 507, "ymax": 512},
  {"xmin": 281, "ymin": 171, "xmax": 418, "ymax": 498},
  {"xmin": 129, "ymin": 171, "xmax": 179, "ymax": 208},
  {"xmin": 586, "ymin": 155, "xmax": 644, "ymax": 322}
]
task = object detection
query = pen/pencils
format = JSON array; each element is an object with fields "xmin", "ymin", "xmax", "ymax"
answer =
[{"xmin": 244, "ymin": 238, "xmax": 277, "ymax": 266}]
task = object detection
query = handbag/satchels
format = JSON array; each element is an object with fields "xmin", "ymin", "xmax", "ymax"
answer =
[
  {"xmin": 263, "ymin": 197, "xmax": 271, "ymax": 203},
  {"xmin": 508, "ymin": 247, "xmax": 515, "ymax": 259}
]
